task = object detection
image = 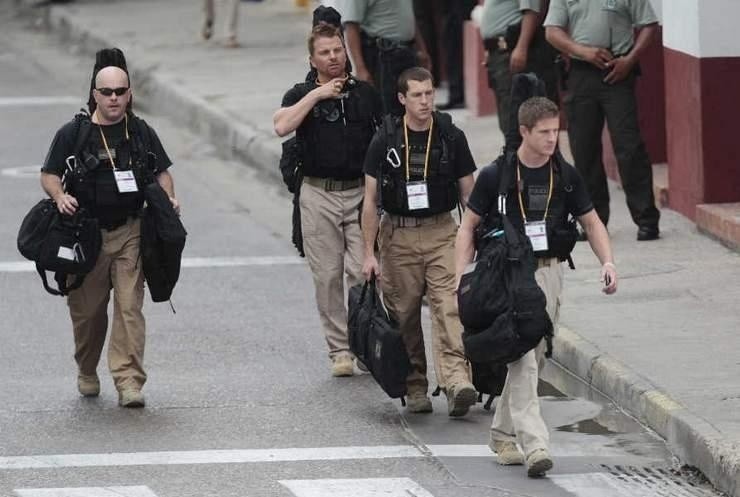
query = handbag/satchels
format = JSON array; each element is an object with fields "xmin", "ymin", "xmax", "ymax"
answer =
[
  {"xmin": 457, "ymin": 213, "xmax": 554, "ymax": 410},
  {"xmin": 280, "ymin": 135, "xmax": 300, "ymax": 194},
  {"xmin": 347, "ymin": 268, "xmax": 410, "ymax": 407},
  {"xmin": 141, "ymin": 180, "xmax": 187, "ymax": 303},
  {"xmin": 17, "ymin": 154, "xmax": 102, "ymax": 298}
]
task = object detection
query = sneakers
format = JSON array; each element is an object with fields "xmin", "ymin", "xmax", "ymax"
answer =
[
  {"xmin": 528, "ymin": 449, "xmax": 554, "ymax": 478},
  {"xmin": 638, "ymin": 225, "xmax": 659, "ymax": 240},
  {"xmin": 119, "ymin": 389, "xmax": 145, "ymax": 408},
  {"xmin": 332, "ymin": 355, "xmax": 353, "ymax": 376},
  {"xmin": 488, "ymin": 440, "xmax": 525, "ymax": 465},
  {"xmin": 407, "ymin": 391, "xmax": 433, "ymax": 413},
  {"xmin": 447, "ymin": 376, "xmax": 478, "ymax": 417},
  {"xmin": 77, "ymin": 375, "xmax": 100, "ymax": 397}
]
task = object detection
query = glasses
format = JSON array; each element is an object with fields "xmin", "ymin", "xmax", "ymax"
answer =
[{"xmin": 96, "ymin": 87, "xmax": 129, "ymax": 95}]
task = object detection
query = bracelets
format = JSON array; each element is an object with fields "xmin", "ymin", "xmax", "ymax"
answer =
[{"xmin": 602, "ymin": 262, "xmax": 616, "ymax": 270}]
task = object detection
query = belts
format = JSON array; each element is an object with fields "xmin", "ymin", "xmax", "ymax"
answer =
[
  {"xmin": 400, "ymin": 39, "xmax": 416, "ymax": 47},
  {"xmin": 98, "ymin": 213, "xmax": 137, "ymax": 231},
  {"xmin": 483, "ymin": 36, "xmax": 509, "ymax": 53},
  {"xmin": 390, "ymin": 211, "xmax": 452, "ymax": 227},
  {"xmin": 302, "ymin": 177, "xmax": 366, "ymax": 191}
]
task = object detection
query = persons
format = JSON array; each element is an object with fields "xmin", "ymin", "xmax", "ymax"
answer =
[
  {"xmin": 543, "ymin": 1, "xmax": 661, "ymax": 241},
  {"xmin": 412, "ymin": 0, "xmax": 479, "ymax": 110},
  {"xmin": 40, "ymin": 65, "xmax": 181, "ymax": 408},
  {"xmin": 361, "ymin": 66, "xmax": 478, "ymax": 417},
  {"xmin": 340, "ymin": 0, "xmax": 432, "ymax": 88},
  {"xmin": 454, "ymin": 96, "xmax": 619, "ymax": 479},
  {"xmin": 277, "ymin": 19, "xmax": 385, "ymax": 379},
  {"xmin": 480, "ymin": 0, "xmax": 560, "ymax": 151},
  {"xmin": 201, "ymin": 0, "xmax": 242, "ymax": 49}
]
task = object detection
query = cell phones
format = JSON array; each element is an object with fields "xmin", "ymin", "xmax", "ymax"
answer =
[{"xmin": 605, "ymin": 271, "xmax": 609, "ymax": 285}]
